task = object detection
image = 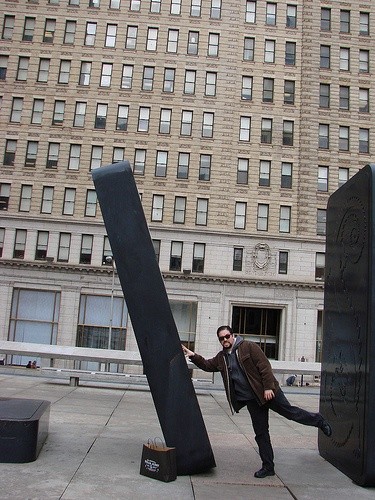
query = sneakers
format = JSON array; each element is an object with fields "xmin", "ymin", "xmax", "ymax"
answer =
[
  {"xmin": 254, "ymin": 466, "xmax": 275, "ymax": 478},
  {"xmin": 315, "ymin": 413, "xmax": 334, "ymax": 437}
]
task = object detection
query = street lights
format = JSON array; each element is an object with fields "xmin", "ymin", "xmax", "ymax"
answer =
[{"xmin": 105, "ymin": 257, "xmax": 116, "ymax": 349}]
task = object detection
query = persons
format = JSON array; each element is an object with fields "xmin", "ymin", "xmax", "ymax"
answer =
[
  {"xmin": 181, "ymin": 326, "xmax": 331, "ymax": 478},
  {"xmin": 26, "ymin": 361, "xmax": 37, "ymax": 369}
]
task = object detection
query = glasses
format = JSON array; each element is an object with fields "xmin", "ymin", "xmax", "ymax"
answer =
[{"xmin": 219, "ymin": 333, "xmax": 232, "ymax": 341}]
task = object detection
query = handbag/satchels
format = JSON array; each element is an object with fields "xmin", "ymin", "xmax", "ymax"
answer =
[{"xmin": 140, "ymin": 436, "xmax": 178, "ymax": 483}]
